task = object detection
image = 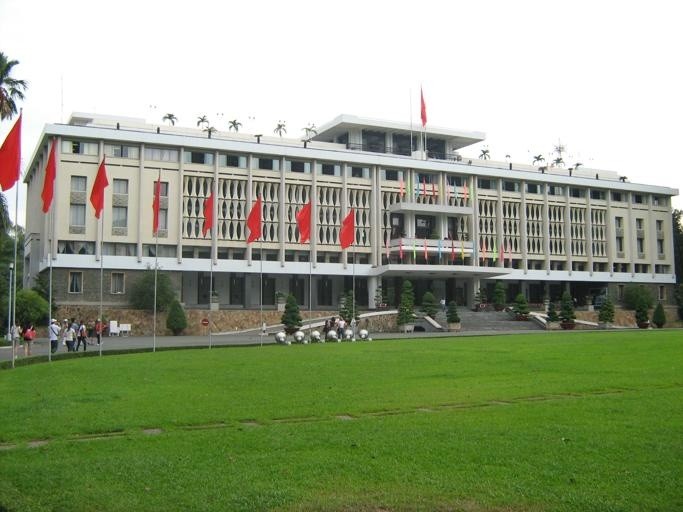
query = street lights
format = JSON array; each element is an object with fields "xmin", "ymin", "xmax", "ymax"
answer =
[{"xmin": 6, "ymin": 263, "xmax": 14, "ymax": 340}]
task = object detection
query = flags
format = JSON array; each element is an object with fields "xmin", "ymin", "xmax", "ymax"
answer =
[
  {"xmin": 385, "ymin": 172, "xmax": 513, "ymax": 267},
  {"xmin": 419, "ymin": 89, "xmax": 429, "ymax": 128},
  {"xmin": 297, "ymin": 202, "xmax": 311, "ymax": 245},
  {"xmin": 247, "ymin": 194, "xmax": 262, "ymax": 245},
  {"xmin": 40, "ymin": 144, "xmax": 58, "ymax": 215},
  {"xmin": 151, "ymin": 178, "xmax": 160, "ymax": 237},
  {"xmin": 202, "ymin": 192, "xmax": 213, "ymax": 237},
  {"xmin": 339, "ymin": 211, "xmax": 356, "ymax": 252},
  {"xmin": 89, "ymin": 159, "xmax": 110, "ymax": 220},
  {"xmin": 1, "ymin": 117, "xmax": 23, "ymax": 193}
]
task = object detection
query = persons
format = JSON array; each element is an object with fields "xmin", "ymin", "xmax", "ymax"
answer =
[
  {"xmin": 11, "ymin": 316, "xmax": 105, "ymax": 361},
  {"xmin": 336, "ymin": 317, "xmax": 347, "ymax": 340},
  {"xmin": 329, "ymin": 316, "xmax": 337, "ymax": 331},
  {"xmin": 572, "ymin": 296, "xmax": 578, "ymax": 308},
  {"xmin": 439, "ymin": 297, "xmax": 445, "ymax": 313},
  {"xmin": 325, "ymin": 319, "xmax": 330, "ymax": 340},
  {"xmin": 334, "ymin": 316, "xmax": 347, "ymax": 338}
]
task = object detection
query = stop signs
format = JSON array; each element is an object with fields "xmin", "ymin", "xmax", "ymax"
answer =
[{"xmin": 202, "ymin": 318, "xmax": 208, "ymax": 326}]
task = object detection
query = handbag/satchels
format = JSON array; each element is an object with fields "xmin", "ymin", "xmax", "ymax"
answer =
[
  {"xmin": 81, "ymin": 331, "xmax": 88, "ymax": 337},
  {"xmin": 72, "ymin": 332, "xmax": 76, "ymax": 339}
]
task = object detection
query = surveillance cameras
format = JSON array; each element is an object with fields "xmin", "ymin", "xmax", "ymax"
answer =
[{"xmin": 9, "ymin": 262, "xmax": 13, "ymax": 269}]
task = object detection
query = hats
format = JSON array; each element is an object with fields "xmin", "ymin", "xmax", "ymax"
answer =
[
  {"xmin": 97, "ymin": 319, "xmax": 102, "ymax": 322},
  {"xmin": 63, "ymin": 319, "xmax": 68, "ymax": 323},
  {"xmin": 51, "ymin": 319, "xmax": 57, "ymax": 323}
]
